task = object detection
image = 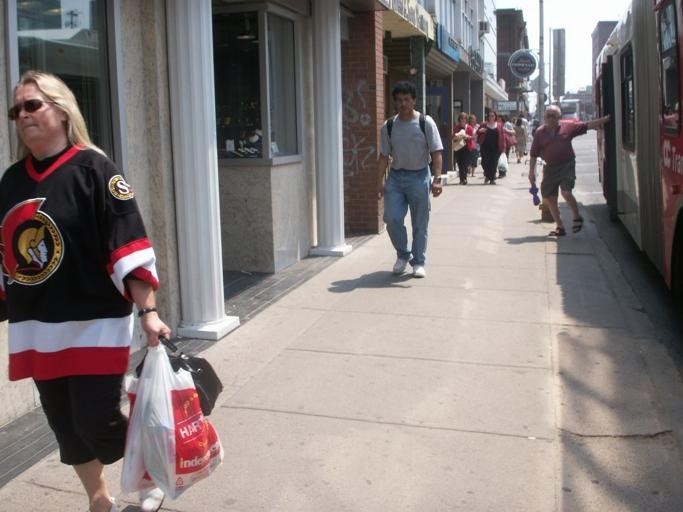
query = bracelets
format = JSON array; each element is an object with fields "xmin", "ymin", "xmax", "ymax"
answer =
[{"xmin": 137, "ymin": 308, "xmax": 157, "ymax": 316}]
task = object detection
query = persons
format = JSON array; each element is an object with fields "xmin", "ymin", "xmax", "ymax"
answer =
[
  {"xmin": 0, "ymin": 69, "xmax": 171, "ymax": 512},
  {"xmin": 374, "ymin": 80, "xmax": 443, "ymax": 278},
  {"xmin": 452, "ymin": 109, "xmax": 539, "ymax": 185},
  {"xmin": 528, "ymin": 105, "xmax": 610, "ymax": 236}
]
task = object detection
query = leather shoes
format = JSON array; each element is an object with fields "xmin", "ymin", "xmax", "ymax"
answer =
[{"xmin": 141, "ymin": 486, "xmax": 164, "ymax": 511}]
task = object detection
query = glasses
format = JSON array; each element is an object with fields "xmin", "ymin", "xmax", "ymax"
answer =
[{"xmin": 8, "ymin": 99, "xmax": 55, "ymax": 120}]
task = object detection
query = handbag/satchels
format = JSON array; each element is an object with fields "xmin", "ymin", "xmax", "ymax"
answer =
[
  {"xmin": 121, "ymin": 335, "xmax": 223, "ymax": 500},
  {"xmin": 453, "ymin": 137, "xmax": 466, "ymax": 151}
]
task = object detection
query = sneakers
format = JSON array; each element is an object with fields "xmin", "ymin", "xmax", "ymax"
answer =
[{"xmin": 393, "ymin": 258, "xmax": 426, "ymax": 278}]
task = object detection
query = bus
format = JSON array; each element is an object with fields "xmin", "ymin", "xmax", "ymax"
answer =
[
  {"xmin": 560, "ymin": 99, "xmax": 582, "ymax": 120},
  {"xmin": 593, "ymin": 0, "xmax": 683, "ymax": 341}
]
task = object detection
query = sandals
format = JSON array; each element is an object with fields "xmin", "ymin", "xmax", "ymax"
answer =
[
  {"xmin": 573, "ymin": 217, "xmax": 583, "ymax": 233},
  {"xmin": 549, "ymin": 227, "xmax": 565, "ymax": 236}
]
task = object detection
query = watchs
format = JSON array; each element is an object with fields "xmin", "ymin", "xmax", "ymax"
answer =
[{"xmin": 434, "ymin": 176, "xmax": 442, "ymax": 182}]
